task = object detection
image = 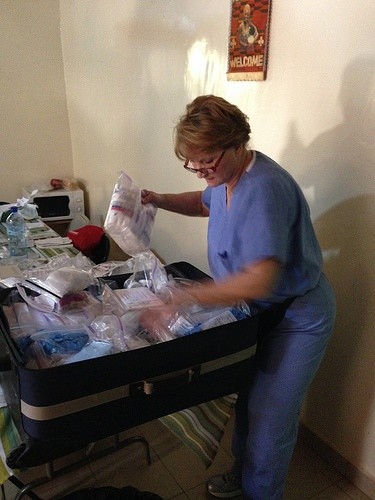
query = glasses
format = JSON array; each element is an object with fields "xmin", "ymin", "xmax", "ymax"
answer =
[{"xmin": 183, "ymin": 149, "xmax": 227, "ymax": 174}]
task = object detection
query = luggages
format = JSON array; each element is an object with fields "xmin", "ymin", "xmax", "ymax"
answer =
[{"xmin": 0, "ymin": 260, "xmax": 264, "ymax": 472}]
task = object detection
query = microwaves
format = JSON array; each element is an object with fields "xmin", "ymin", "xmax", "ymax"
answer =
[{"xmin": 28, "ymin": 186, "xmax": 86, "ymax": 221}]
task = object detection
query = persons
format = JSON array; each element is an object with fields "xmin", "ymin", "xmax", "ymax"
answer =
[{"xmin": 138, "ymin": 96, "xmax": 334, "ymax": 500}]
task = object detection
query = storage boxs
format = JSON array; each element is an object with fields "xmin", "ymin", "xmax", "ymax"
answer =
[{"xmin": 0, "ymin": 259, "xmax": 260, "ymax": 443}]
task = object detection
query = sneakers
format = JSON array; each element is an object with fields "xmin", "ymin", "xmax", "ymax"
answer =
[{"xmin": 206, "ymin": 468, "xmax": 244, "ymax": 498}]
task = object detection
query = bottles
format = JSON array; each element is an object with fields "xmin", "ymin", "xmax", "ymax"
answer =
[{"xmin": 6, "ymin": 206, "xmax": 27, "ymax": 257}]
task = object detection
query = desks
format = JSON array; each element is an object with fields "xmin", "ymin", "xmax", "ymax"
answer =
[{"xmin": 0, "ymin": 217, "xmax": 162, "ymax": 500}]
